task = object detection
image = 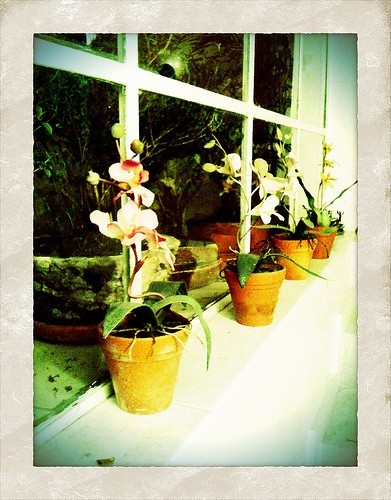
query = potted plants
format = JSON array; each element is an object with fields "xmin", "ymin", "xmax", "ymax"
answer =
[
  {"xmin": 143, "ymin": 153, "xmax": 222, "ymax": 290},
  {"xmin": 34, "ymin": 106, "xmax": 123, "ymax": 347}
]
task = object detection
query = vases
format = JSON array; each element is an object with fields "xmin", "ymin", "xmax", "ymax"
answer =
[
  {"xmin": 197, "ymin": 221, "xmax": 269, "ymax": 254},
  {"xmin": 98, "ymin": 315, "xmax": 189, "ymax": 417},
  {"xmin": 305, "ymin": 226, "xmax": 338, "ymax": 259},
  {"xmin": 225, "ymin": 260, "xmax": 286, "ymax": 327},
  {"xmin": 272, "ymin": 233, "xmax": 319, "ymax": 280}
]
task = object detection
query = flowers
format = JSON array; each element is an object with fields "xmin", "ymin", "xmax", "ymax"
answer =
[
  {"xmin": 85, "ymin": 123, "xmax": 212, "ymax": 370},
  {"xmin": 204, "ymin": 127, "xmax": 357, "ymax": 288}
]
